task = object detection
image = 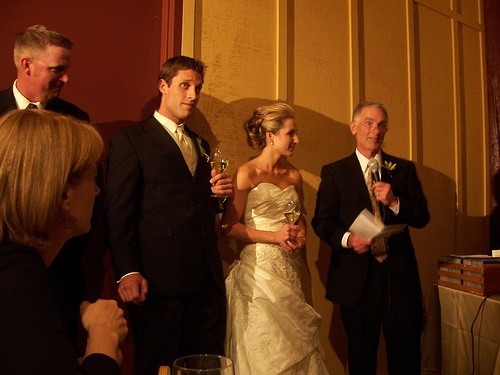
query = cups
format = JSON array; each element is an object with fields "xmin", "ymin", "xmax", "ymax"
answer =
[{"xmin": 172, "ymin": 354, "xmax": 234, "ymax": 375}]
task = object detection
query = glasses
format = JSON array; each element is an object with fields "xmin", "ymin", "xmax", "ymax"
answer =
[{"xmin": 355, "ymin": 120, "xmax": 389, "ymax": 133}]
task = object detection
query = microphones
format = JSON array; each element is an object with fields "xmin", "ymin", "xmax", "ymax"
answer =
[{"xmin": 366, "ymin": 158, "xmax": 380, "ymax": 182}]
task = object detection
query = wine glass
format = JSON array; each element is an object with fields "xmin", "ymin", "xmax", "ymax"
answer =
[
  {"xmin": 209, "ymin": 146, "xmax": 229, "ymax": 198},
  {"xmin": 284, "ymin": 199, "xmax": 301, "ymax": 245}
]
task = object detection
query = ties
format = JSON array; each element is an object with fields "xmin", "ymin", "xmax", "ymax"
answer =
[
  {"xmin": 367, "ymin": 163, "xmax": 389, "ymax": 263},
  {"xmin": 25, "ymin": 103, "xmax": 39, "ymax": 110},
  {"xmin": 176, "ymin": 126, "xmax": 198, "ymax": 177}
]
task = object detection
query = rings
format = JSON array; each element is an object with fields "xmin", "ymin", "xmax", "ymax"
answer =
[
  {"xmin": 377, "ymin": 191, "xmax": 380, "ymax": 197},
  {"xmin": 224, "ymin": 184, "xmax": 227, "ymax": 190}
]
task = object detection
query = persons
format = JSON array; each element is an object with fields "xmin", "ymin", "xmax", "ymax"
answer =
[
  {"xmin": 104, "ymin": 55, "xmax": 234, "ymax": 375},
  {"xmin": 0, "ymin": 23, "xmax": 91, "ymax": 354},
  {"xmin": 0, "ymin": 109, "xmax": 129, "ymax": 375},
  {"xmin": 219, "ymin": 102, "xmax": 331, "ymax": 375},
  {"xmin": 309, "ymin": 100, "xmax": 431, "ymax": 375}
]
